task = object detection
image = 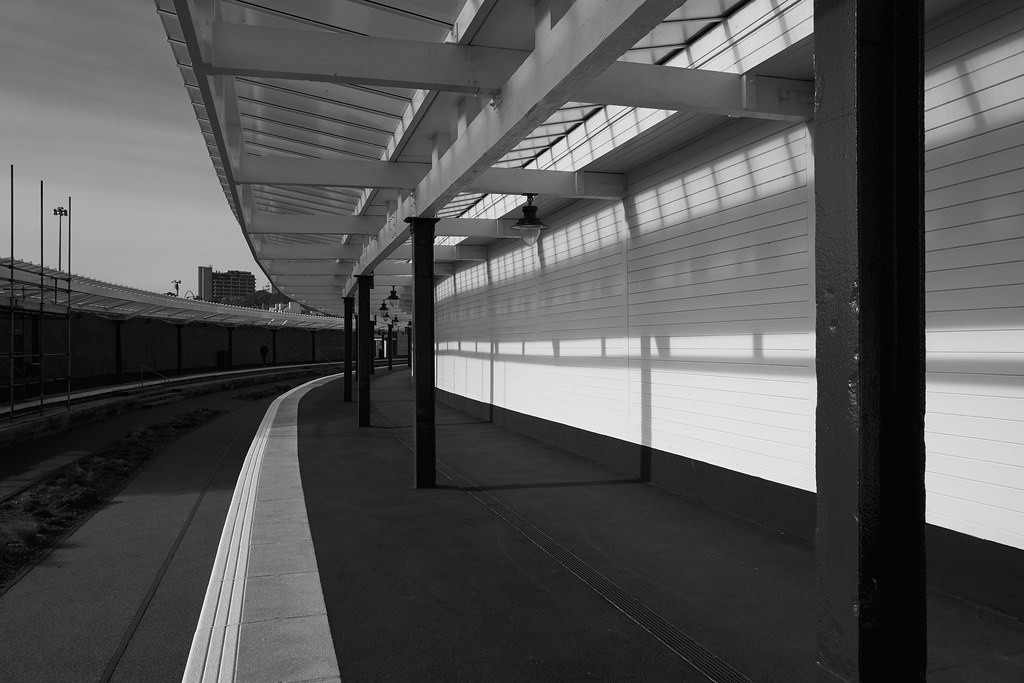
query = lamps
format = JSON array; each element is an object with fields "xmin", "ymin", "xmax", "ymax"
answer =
[
  {"xmin": 393, "ymin": 317, "xmax": 399, "ymax": 326},
  {"xmin": 386, "ymin": 286, "xmax": 400, "ymax": 307},
  {"xmin": 407, "ymin": 320, "xmax": 412, "ymax": 326},
  {"xmin": 379, "ymin": 300, "xmax": 388, "ymax": 311},
  {"xmin": 381, "ymin": 312, "xmax": 390, "ymax": 322},
  {"xmin": 511, "ymin": 193, "xmax": 548, "ymax": 248}
]
